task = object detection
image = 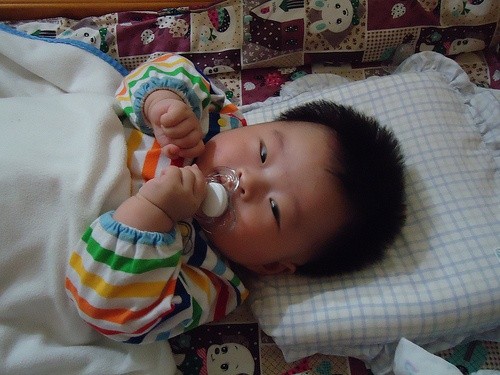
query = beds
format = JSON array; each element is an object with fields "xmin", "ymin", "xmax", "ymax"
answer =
[{"xmin": 0, "ymin": 0, "xmax": 500, "ymax": 375}]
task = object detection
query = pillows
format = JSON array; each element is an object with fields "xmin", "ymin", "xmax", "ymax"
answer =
[{"xmin": 235, "ymin": 50, "xmax": 500, "ymax": 373}]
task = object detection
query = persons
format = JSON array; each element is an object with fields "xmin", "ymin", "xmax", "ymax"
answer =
[{"xmin": 64, "ymin": 52, "xmax": 409, "ymax": 344}]
